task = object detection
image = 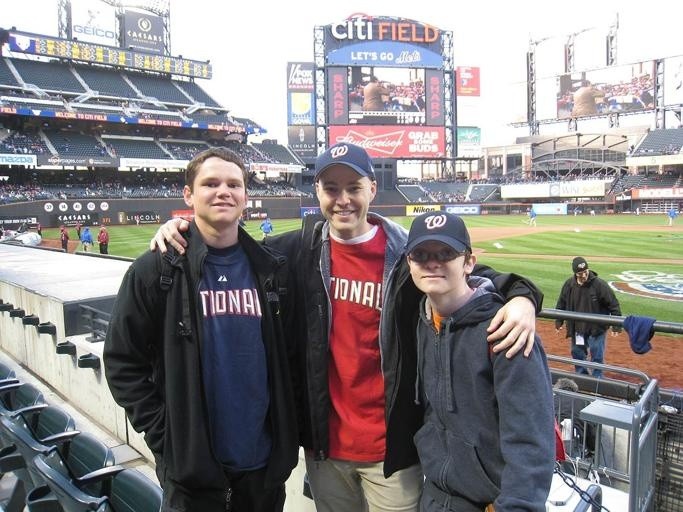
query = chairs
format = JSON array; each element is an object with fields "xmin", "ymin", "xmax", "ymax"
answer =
[
  {"xmin": 610, "ymin": 124, "xmax": 682, "ymax": 191},
  {"xmin": 0, "ymin": 32, "xmax": 306, "ymax": 207},
  {"xmin": 395, "ymin": 177, "xmax": 500, "ymax": 202},
  {"xmin": 0, "ymin": 356, "xmax": 177, "ymax": 512}
]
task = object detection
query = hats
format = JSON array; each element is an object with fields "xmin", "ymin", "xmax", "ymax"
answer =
[
  {"xmin": 315, "ymin": 143, "xmax": 375, "ymax": 182},
  {"xmin": 573, "ymin": 258, "xmax": 588, "ymax": 272},
  {"xmin": 405, "ymin": 212, "xmax": 471, "ymax": 256}
]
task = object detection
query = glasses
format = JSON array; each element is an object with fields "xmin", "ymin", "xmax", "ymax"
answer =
[{"xmin": 409, "ymin": 249, "xmax": 466, "ymax": 262}]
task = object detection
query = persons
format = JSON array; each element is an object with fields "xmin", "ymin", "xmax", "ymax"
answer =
[
  {"xmin": 149, "ymin": 142, "xmax": 543, "ymax": 512},
  {"xmin": 554, "ymin": 257, "xmax": 623, "ymax": 381},
  {"xmin": 348, "ymin": 76, "xmax": 425, "ymax": 111},
  {"xmin": 558, "ymin": 76, "xmax": 652, "ymax": 118},
  {"xmin": 402, "ymin": 209, "xmax": 556, "ymax": 512},
  {"xmin": 101, "ymin": 143, "xmax": 304, "ymax": 512},
  {"xmin": 551, "ymin": 378, "xmax": 603, "ymax": 460},
  {"xmin": 1, "ymin": 132, "xmax": 683, "ymax": 257}
]
